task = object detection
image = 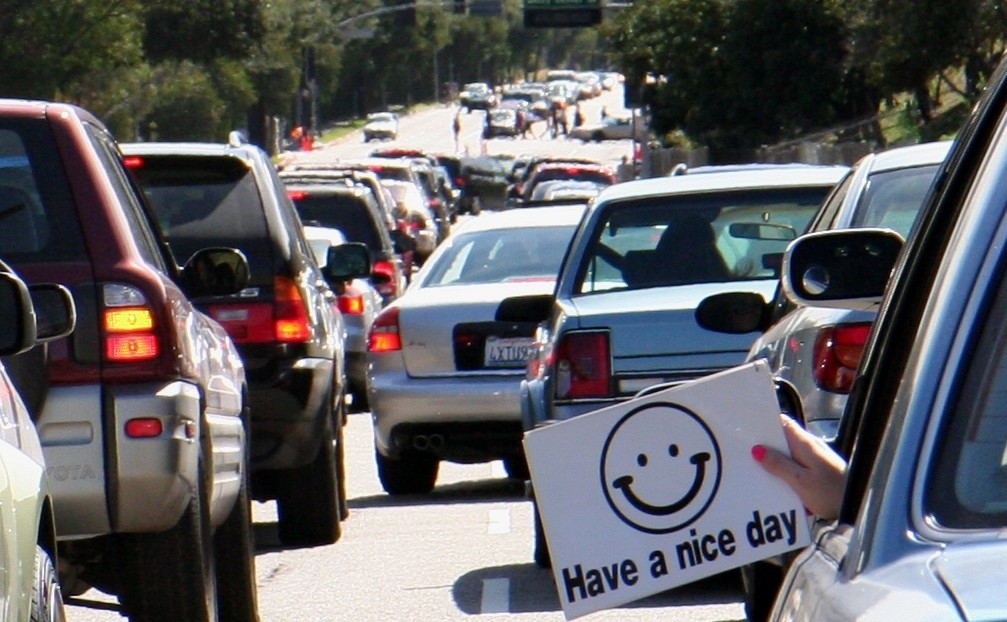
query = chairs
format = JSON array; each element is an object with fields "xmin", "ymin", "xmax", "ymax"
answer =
[
  {"xmin": 621, "ymin": 245, "xmax": 731, "ymax": 287},
  {"xmin": 0, "ymin": 185, "xmax": 45, "ymax": 255},
  {"xmin": 166, "ymin": 212, "xmax": 227, "ymax": 261}
]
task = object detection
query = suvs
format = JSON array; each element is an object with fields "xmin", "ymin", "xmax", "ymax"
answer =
[
  {"xmin": 757, "ymin": 42, "xmax": 1007, "ymax": 622},
  {"xmin": 112, "ymin": 131, "xmax": 372, "ymax": 551},
  {"xmin": 694, "ymin": 137, "xmax": 959, "ymax": 622},
  {"xmin": 0, "ymin": 95, "xmax": 262, "ymax": 622},
  {"xmin": 491, "ymin": 161, "xmax": 859, "ymax": 580}
]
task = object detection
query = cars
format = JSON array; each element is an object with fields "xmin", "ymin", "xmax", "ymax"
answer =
[
  {"xmin": 279, "ymin": 223, "xmax": 387, "ymax": 418},
  {"xmin": 0, "ymin": 259, "xmax": 78, "ymax": 622},
  {"xmin": 274, "ymin": 66, "xmax": 644, "ymax": 304},
  {"xmin": 363, "ymin": 204, "xmax": 616, "ymax": 495}
]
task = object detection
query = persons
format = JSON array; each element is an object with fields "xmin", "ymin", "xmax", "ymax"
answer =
[
  {"xmin": 513, "ymin": 101, "xmax": 584, "ymax": 140},
  {"xmin": 752, "ymin": 413, "xmax": 849, "ymax": 521}
]
